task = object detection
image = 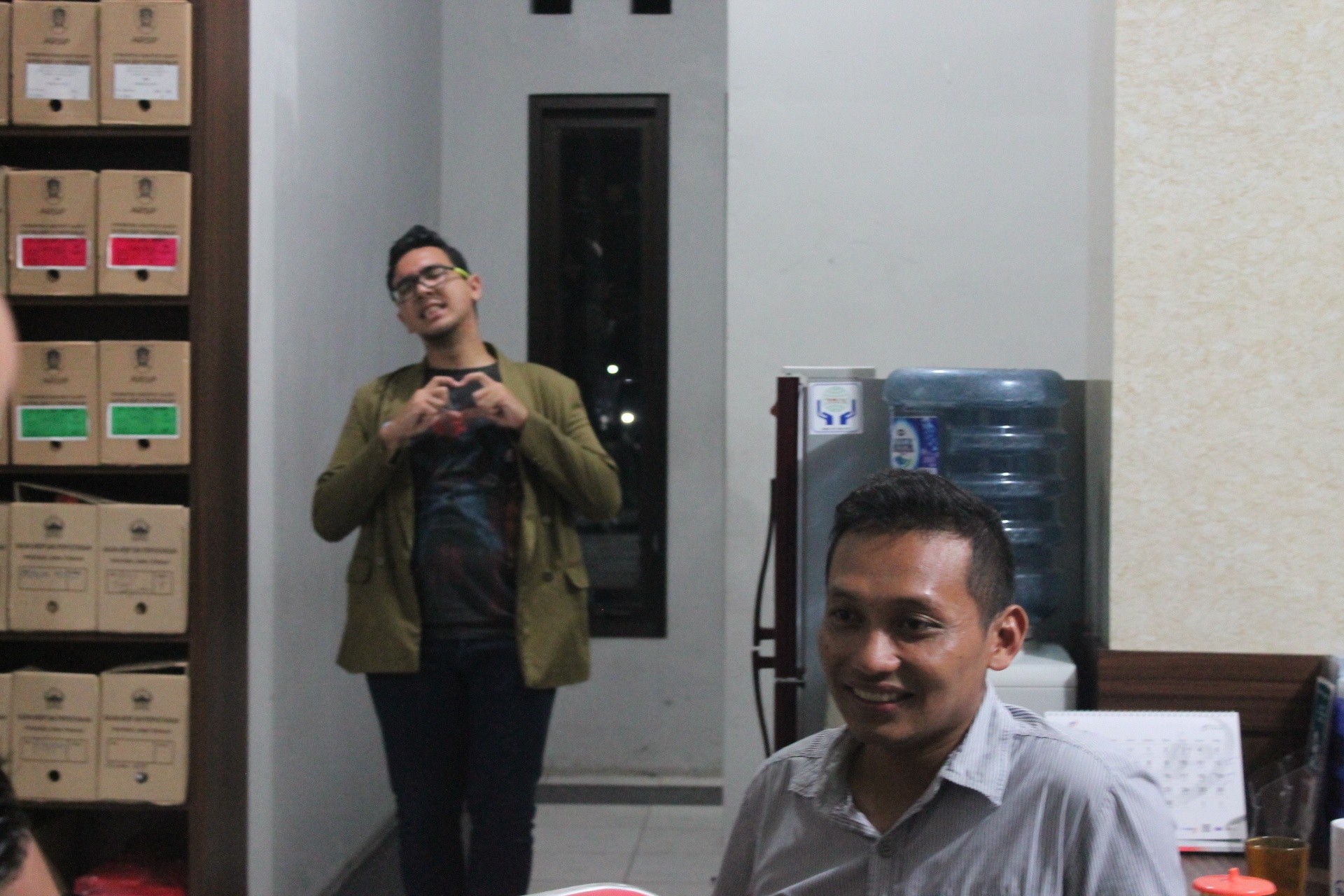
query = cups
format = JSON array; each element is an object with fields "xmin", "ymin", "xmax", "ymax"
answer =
[{"xmin": 1245, "ymin": 836, "xmax": 1310, "ymax": 896}]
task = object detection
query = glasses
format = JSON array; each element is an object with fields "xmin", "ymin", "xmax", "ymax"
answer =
[{"xmin": 391, "ymin": 263, "xmax": 471, "ymax": 304}]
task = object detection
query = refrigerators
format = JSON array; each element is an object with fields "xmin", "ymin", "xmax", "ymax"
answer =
[{"xmin": 742, "ymin": 362, "xmax": 1083, "ymax": 767}]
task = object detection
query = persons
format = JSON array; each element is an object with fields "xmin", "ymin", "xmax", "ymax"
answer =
[
  {"xmin": 706, "ymin": 467, "xmax": 1198, "ymax": 896},
  {"xmin": 303, "ymin": 223, "xmax": 622, "ymax": 896}
]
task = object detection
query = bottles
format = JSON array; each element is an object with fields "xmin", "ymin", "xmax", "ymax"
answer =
[{"xmin": 883, "ymin": 367, "xmax": 1065, "ymax": 639}]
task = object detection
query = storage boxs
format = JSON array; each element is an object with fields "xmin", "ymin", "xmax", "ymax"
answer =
[{"xmin": 1, "ymin": 1, "xmax": 202, "ymax": 805}]
